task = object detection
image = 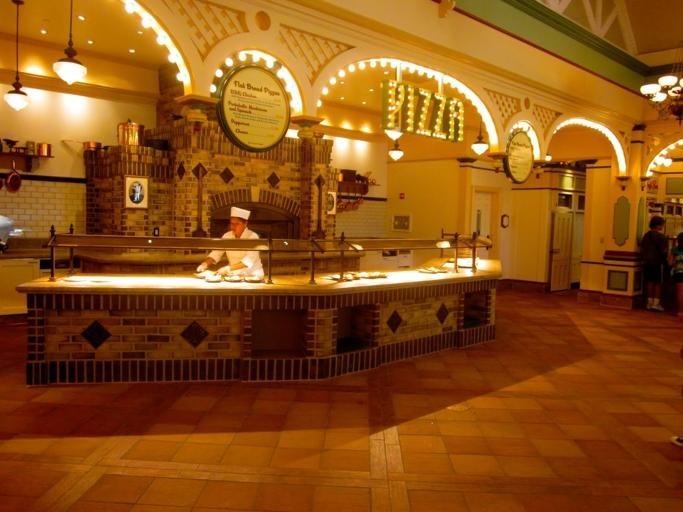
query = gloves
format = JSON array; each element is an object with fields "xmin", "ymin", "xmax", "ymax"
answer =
[
  {"xmin": 218, "ymin": 265, "xmax": 230, "ymax": 272},
  {"xmin": 197, "ymin": 262, "xmax": 208, "ymax": 272}
]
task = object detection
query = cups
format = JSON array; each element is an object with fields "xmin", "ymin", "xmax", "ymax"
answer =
[{"xmin": 37, "ymin": 143, "xmax": 51, "ymax": 157}]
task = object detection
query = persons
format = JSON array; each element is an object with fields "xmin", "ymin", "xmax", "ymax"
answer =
[
  {"xmin": 640, "ymin": 215, "xmax": 668, "ymax": 311},
  {"xmin": 667, "ymin": 231, "xmax": 682, "ymax": 316},
  {"xmin": 195, "ymin": 207, "xmax": 264, "ymax": 276},
  {"xmin": 129, "ymin": 181, "xmax": 144, "ymax": 204}
]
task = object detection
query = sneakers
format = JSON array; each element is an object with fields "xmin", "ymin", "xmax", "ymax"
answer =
[{"xmin": 647, "ymin": 304, "xmax": 664, "ymax": 311}]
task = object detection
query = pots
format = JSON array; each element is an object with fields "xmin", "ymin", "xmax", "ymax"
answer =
[
  {"xmin": 5, "ymin": 160, "xmax": 22, "ymax": 193},
  {"xmin": 117, "ymin": 119, "xmax": 145, "ymax": 146}
]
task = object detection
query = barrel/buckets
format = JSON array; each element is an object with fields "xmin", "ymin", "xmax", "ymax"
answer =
[
  {"xmin": 117, "ymin": 122, "xmax": 145, "ymax": 146},
  {"xmin": 38, "ymin": 143, "xmax": 52, "ymax": 156},
  {"xmin": 25, "ymin": 141, "xmax": 35, "ymax": 154},
  {"xmin": 84, "ymin": 142, "xmax": 101, "ymax": 151}
]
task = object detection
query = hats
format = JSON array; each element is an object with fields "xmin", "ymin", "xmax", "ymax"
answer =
[{"xmin": 230, "ymin": 207, "xmax": 251, "ymax": 220}]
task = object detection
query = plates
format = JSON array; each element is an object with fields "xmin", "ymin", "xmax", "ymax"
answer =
[
  {"xmin": 328, "ymin": 272, "xmax": 387, "ymax": 281},
  {"xmin": 417, "ymin": 267, "xmax": 447, "ymax": 273},
  {"xmin": 194, "ymin": 271, "xmax": 264, "ymax": 283}
]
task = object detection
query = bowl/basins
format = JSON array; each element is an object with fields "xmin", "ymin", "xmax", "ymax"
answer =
[
  {"xmin": 83, "ymin": 141, "xmax": 102, "ymax": 152},
  {"xmin": 144, "ymin": 138, "xmax": 169, "ymax": 150}
]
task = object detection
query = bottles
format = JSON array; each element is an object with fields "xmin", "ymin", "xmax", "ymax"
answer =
[{"xmin": 153, "ymin": 226, "xmax": 159, "ymax": 236}]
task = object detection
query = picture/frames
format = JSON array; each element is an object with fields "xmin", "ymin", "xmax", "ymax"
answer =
[{"xmin": 123, "ymin": 174, "xmax": 149, "ymax": 211}]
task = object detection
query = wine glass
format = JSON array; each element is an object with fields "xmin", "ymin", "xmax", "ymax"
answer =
[{"xmin": 4, "ymin": 138, "xmax": 19, "ymax": 152}]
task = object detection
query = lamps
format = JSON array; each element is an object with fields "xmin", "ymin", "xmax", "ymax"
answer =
[
  {"xmin": 469, "ymin": 117, "xmax": 489, "ymax": 157},
  {"xmin": 388, "ymin": 139, "xmax": 405, "ymax": 162},
  {"xmin": 52, "ymin": 1, "xmax": 88, "ymax": 86},
  {"xmin": 639, "ymin": 69, "xmax": 683, "ymax": 125},
  {"xmin": 3, "ymin": 0, "xmax": 30, "ymax": 112}
]
